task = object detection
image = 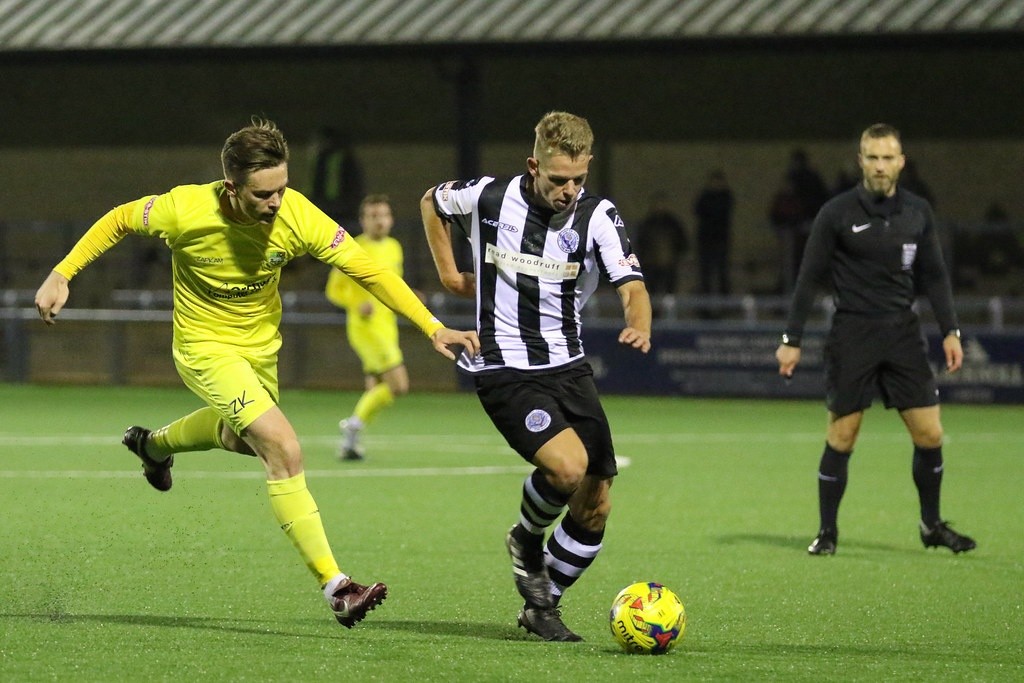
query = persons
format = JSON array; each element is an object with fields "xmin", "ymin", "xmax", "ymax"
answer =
[
  {"xmin": 770, "ymin": 147, "xmax": 938, "ymax": 292},
  {"xmin": 307, "ymin": 122, "xmax": 362, "ymax": 213},
  {"xmin": 419, "ymin": 111, "xmax": 652, "ymax": 640},
  {"xmin": 324, "ymin": 193, "xmax": 409, "ymax": 460},
  {"xmin": 771, "ymin": 122, "xmax": 976, "ymax": 555},
  {"xmin": 630, "ymin": 191, "xmax": 688, "ymax": 302},
  {"xmin": 689, "ymin": 166, "xmax": 733, "ymax": 297},
  {"xmin": 33, "ymin": 110, "xmax": 482, "ymax": 627}
]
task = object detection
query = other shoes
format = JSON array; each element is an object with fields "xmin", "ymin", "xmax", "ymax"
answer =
[{"xmin": 335, "ymin": 418, "xmax": 365, "ymax": 459}]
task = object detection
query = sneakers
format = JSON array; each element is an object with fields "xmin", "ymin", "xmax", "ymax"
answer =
[
  {"xmin": 328, "ymin": 582, "xmax": 387, "ymax": 629},
  {"xmin": 517, "ymin": 601, "xmax": 582, "ymax": 642},
  {"xmin": 122, "ymin": 425, "xmax": 174, "ymax": 491},
  {"xmin": 808, "ymin": 527, "xmax": 838, "ymax": 555},
  {"xmin": 920, "ymin": 521, "xmax": 975, "ymax": 552},
  {"xmin": 506, "ymin": 524, "xmax": 553, "ymax": 610}
]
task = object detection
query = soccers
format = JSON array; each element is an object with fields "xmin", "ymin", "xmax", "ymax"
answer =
[{"xmin": 609, "ymin": 582, "xmax": 687, "ymax": 655}]
